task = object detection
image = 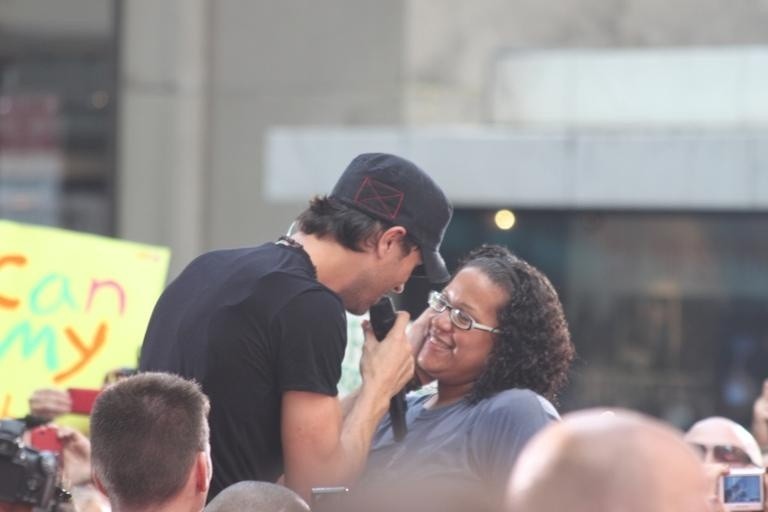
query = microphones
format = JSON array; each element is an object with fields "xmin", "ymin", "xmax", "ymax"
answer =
[{"xmin": 369, "ymin": 295, "xmax": 408, "ymax": 442}]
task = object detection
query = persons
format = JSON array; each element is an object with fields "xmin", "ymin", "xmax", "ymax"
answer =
[
  {"xmin": 138, "ymin": 152, "xmax": 451, "ymax": 512},
  {"xmin": 505, "ymin": 376, "xmax": 768, "ymax": 510},
  {"xmin": 310, "ymin": 244, "xmax": 574, "ymax": 512},
  {"xmin": 2, "ymin": 368, "xmax": 310, "ymax": 510}
]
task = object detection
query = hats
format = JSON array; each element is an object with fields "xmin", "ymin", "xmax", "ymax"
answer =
[{"xmin": 330, "ymin": 152, "xmax": 455, "ymax": 284}]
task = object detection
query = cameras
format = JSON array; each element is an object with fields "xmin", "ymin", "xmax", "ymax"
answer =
[{"xmin": 719, "ymin": 473, "xmax": 763, "ymax": 511}]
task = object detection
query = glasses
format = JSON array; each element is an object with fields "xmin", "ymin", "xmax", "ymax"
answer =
[
  {"xmin": 425, "ymin": 287, "xmax": 511, "ymax": 336},
  {"xmin": 686, "ymin": 440, "xmax": 765, "ymax": 470}
]
